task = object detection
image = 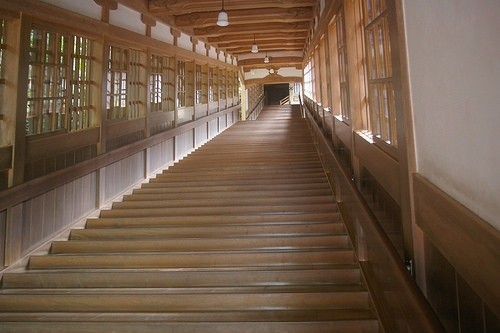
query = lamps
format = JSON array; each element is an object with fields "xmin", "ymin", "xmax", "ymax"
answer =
[
  {"xmin": 250, "ymin": 33, "xmax": 258, "ymax": 53},
  {"xmin": 216, "ymin": 0, "xmax": 229, "ymax": 26},
  {"xmin": 264, "ymin": 50, "xmax": 269, "ymax": 64}
]
